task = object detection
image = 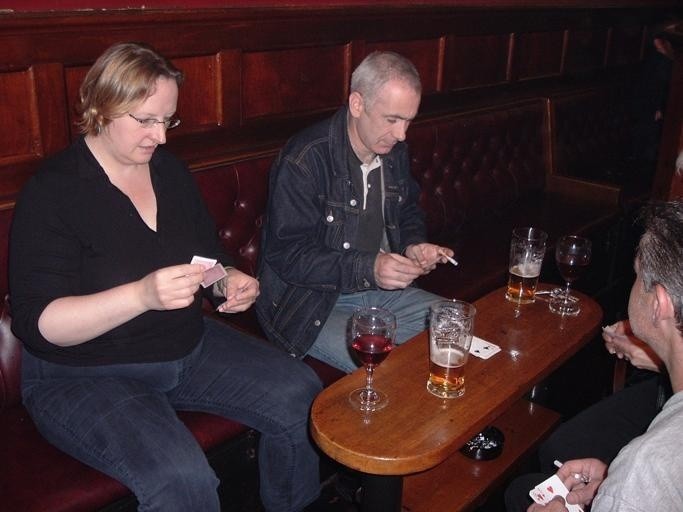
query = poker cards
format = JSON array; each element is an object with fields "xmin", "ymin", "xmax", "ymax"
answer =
[
  {"xmin": 191, "ymin": 255, "xmax": 228, "ymax": 289},
  {"xmin": 454, "ymin": 335, "xmax": 501, "ymax": 361},
  {"xmin": 529, "ymin": 474, "xmax": 585, "ymax": 512},
  {"xmin": 602, "ymin": 325, "xmax": 628, "ymax": 340}
]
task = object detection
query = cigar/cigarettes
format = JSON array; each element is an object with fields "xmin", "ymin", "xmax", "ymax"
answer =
[
  {"xmin": 438, "ymin": 250, "xmax": 459, "ymax": 266},
  {"xmin": 555, "ymin": 460, "xmax": 580, "ymax": 479}
]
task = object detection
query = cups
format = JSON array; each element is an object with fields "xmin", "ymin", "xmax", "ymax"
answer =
[
  {"xmin": 424, "ymin": 299, "xmax": 478, "ymax": 399},
  {"xmin": 504, "ymin": 225, "xmax": 548, "ymax": 304}
]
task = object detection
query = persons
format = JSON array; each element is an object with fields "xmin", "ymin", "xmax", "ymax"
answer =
[
  {"xmin": 501, "ymin": 202, "xmax": 683, "ymax": 512},
  {"xmin": 253, "ymin": 51, "xmax": 448, "ymax": 375},
  {"xmin": 7, "ymin": 42, "xmax": 326, "ymax": 512},
  {"xmin": 536, "ymin": 149, "xmax": 683, "ymax": 469}
]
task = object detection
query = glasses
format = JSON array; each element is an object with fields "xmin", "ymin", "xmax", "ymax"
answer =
[{"xmin": 126, "ymin": 112, "xmax": 183, "ymax": 131}]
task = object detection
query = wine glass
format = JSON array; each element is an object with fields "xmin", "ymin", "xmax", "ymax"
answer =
[
  {"xmin": 544, "ymin": 232, "xmax": 593, "ymax": 317},
  {"xmin": 345, "ymin": 304, "xmax": 398, "ymax": 411}
]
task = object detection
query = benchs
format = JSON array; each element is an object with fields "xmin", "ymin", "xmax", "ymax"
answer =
[{"xmin": 0, "ymin": 93, "xmax": 624, "ymax": 511}]
